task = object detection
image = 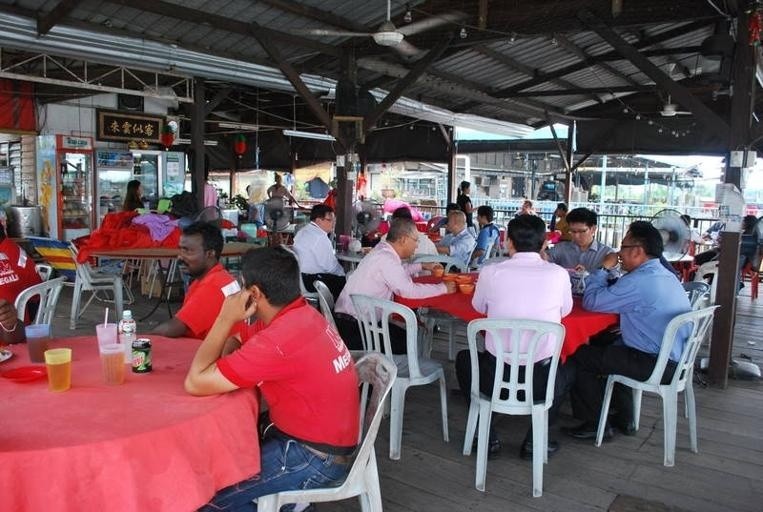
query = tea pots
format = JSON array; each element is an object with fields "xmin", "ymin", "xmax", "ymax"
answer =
[{"xmin": 571, "ymin": 263, "xmax": 590, "ymax": 297}]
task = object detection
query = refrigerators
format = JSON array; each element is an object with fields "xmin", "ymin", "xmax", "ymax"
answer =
[
  {"xmin": 36, "ymin": 135, "xmax": 97, "ymax": 268},
  {"xmin": 95, "ymin": 150, "xmax": 134, "ymax": 232},
  {"xmin": 130, "ymin": 150, "xmax": 163, "ymax": 201}
]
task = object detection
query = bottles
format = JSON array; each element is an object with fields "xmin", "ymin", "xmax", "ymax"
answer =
[{"xmin": 118, "ymin": 310, "xmax": 137, "ymax": 364}]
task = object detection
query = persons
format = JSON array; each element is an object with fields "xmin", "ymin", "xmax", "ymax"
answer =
[
  {"xmin": 514, "ymin": 200, "xmax": 539, "ymax": 218},
  {"xmin": 560, "ymin": 220, "xmax": 692, "ymax": 440},
  {"xmin": 739, "ymin": 215, "xmax": 758, "ymax": 297},
  {"xmin": 246, "ymin": 185, "xmax": 264, "ymax": 226},
  {"xmin": 434, "ymin": 210, "xmax": 476, "ymax": 272},
  {"xmin": 267, "ymin": 175, "xmax": 304, "ymax": 209},
  {"xmin": 380, "ymin": 206, "xmax": 439, "ymax": 257},
  {"xmin": 690, "ymin": 221, "xmax": 722, "ymax": 286},
  {"xmin": 0, "ymin": 299, "xmax": 25, "ymax": 346},
  {"xmin": 469, "ymin": 206, "xmax": 501, "ymax": 269},
  {"xmin": 142, "ymin": 220, "xmax": 250, "ymax": 342},
  {"xmin": 184, "ymin": 246, "xmax": 360, "ymax": 512},
  {"xmin": 333, "ymin": 218, "xmax": 457, "ymax": 355},
  {"xmin": 457, "ymin": 181, "xmax": 478, "ymax": 240},
  {"xmin": 549, "ymin": 203, "xmax": 571, "ymax": 243},
  {"xmin": 204, "ymin": 179, "xmax": 217, "ymax": 221},
  {"xmin": 1, "ymin": 250, "xmax": 30, "ymax": 328},
  {"xmin": 427, "ymin": 203, "xmax": 461, "ymax": 237},
  {"xmin": 543, "ymin": 207, "xmax": 620, "ymax": 280},
  {"xmin": 0, "ymin": 220, "xmax": 43, "ymax": 327},
  {"xmin": 123, "ymin": 180, "xmax": 145, "ymax": 211},
  {"xmin": 680, "ymin": 215, "xmax": 705, "ymax": 259},
  {"xmin": 455, "ymin": 214, "xmax": 574, "ymax": 460},
  {"xmin": 293, "ymin": 204, "xmax": 347, "ymax": 302}
]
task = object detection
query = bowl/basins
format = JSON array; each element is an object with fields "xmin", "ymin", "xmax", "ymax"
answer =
[
  {"xmin": 459, "ymin": 285, "xmax": 474, "ymax": 295},
  {"xmin": 456, "ymin": 275, "xmax": 472, "ymax": 284},
  {"xmin": 432, "ymin": 270, "xmax": 444, "ymax": 278}
]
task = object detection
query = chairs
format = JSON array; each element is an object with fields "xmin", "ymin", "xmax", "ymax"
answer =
[
  {"xmin": 13, "ymin": 224, "xmax": 299, "ymax": 330},
  {"xmin": 252, "ymin": 351, "xmax": 398, "ymax": 512}
]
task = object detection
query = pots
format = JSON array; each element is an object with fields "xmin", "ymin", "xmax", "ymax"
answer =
[{"xmin": 9, "ymin": 200, "xmax": 42, "ymax": 239}]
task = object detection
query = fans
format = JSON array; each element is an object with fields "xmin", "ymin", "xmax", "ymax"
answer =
[
  {"xmin": 642, "ymin": 63, "xmax": 692, "ymax": 116},
  {"xmin": 260, "ymin": 196, "xmax": 293, "ymax": 245},
  {"xmin": 650, "ymin": 209, "xmax": 691, "ymax": 264},
  {"xmin": 308, "ymin": 0, "xmax": 466, "ymax": 58},
  {"xmin": 746, "ymin": 216, "xmax": 763, "ymax": 273}
]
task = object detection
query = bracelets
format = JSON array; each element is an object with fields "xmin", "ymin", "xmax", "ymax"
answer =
[
  {"xmin": 597, "ymin": 265, "xmax": 611, "ymax": 273},
  {"xmin": 0, "ymin": 322, "xmax": 18, "ymax": 332}
]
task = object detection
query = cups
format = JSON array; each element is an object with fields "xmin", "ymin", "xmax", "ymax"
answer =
[
  {"xmin": 44, "ymin": 348, "xmax": 72, "ymax": 393},
  {"xmin": 361, "ymin": 247, "xmax": 371, "ymax": 256},
  {"xmin": 100, "ymin": 344, "xmax": 126, "ymax": 386},
  {"xmin": 25, "ymin": 324, "xmax": 49, "ymax": 364},
  {"xmin": 96, "ymin": 323, "xmax": 117, "ymax": 354},
  {"xmin": 439, "ymin": 228, "xmax": 446, "ymax": 237}
]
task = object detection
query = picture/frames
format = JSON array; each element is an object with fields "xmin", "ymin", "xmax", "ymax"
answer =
[{"xmin": 96, "ymin": 108, "xmax": 166, "ymax": 145}]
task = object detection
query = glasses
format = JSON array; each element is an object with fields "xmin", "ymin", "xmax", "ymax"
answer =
[
  {"xmin": 406, "ymin": 234, "xmax": 420, "ymax": 244},
  {"xmin": 322, "ymin": 217, "xmax": 336, "ymax": 224},
  {"xmin": 566, "ymin": 230, "xmax": 589, "ymax": 235}
]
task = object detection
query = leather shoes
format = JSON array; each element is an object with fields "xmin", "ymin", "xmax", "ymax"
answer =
[
  {"xmin": 521, "ymin": 439, "xmax": 558, "ymax": 460},
  {"xmin": 471, "ymin": 437, "xmax": 501, "ymax": 459},
  {"xmin": 561, "ymin": 423, "xmax": 613, "ymax": 443},
  {"xmin": 612, "ymin": 418, "xmax": 635, "ymax": 437}
]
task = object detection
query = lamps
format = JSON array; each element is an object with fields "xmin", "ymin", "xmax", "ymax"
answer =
[
  {"xmin": 512, "ymin": 150, "xmax": 525, "ymax": 167},
  {"xmin": 506, "ymin": 32, "xmax": 517, "ymax": 46},
  {"xmin": 460, "ymin": 26, "xmax": 467, "ymax": 38},
  {"xmin": 623, "ymin": 106, "xmax": 696, "ymax": 138},
  {"xmin": 403, "ymin": 7, "xmax": 412, "ymax": 21}
]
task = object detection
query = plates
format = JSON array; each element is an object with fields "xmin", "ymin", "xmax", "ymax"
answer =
[
  {"xmin": 0, "ymin": 350, "xmax": 12, "ymax": 362},
  {"xmin": 0, "ymin": 365, "xmax": 47, "ymax": 384},
  {"xmin": 442, "ymin": 274, "xmax": 457, "ymax": 281}
]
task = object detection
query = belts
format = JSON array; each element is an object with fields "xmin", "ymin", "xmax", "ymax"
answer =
[
  {"xmin": 485, "ymin": 352, "xmax": 550, "ymax": 368},
  {"xmin": 301, "ymin": 443, "xmax": 358, "ymax": 469},
  {"xmin": 333, "ymin": 313, "xmax": 356, "ymax": 322}
]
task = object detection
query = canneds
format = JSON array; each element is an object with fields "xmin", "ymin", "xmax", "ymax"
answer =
[{"xmin": 132, "ymin": 338, "xmax": 153, "ymax": 372}]
